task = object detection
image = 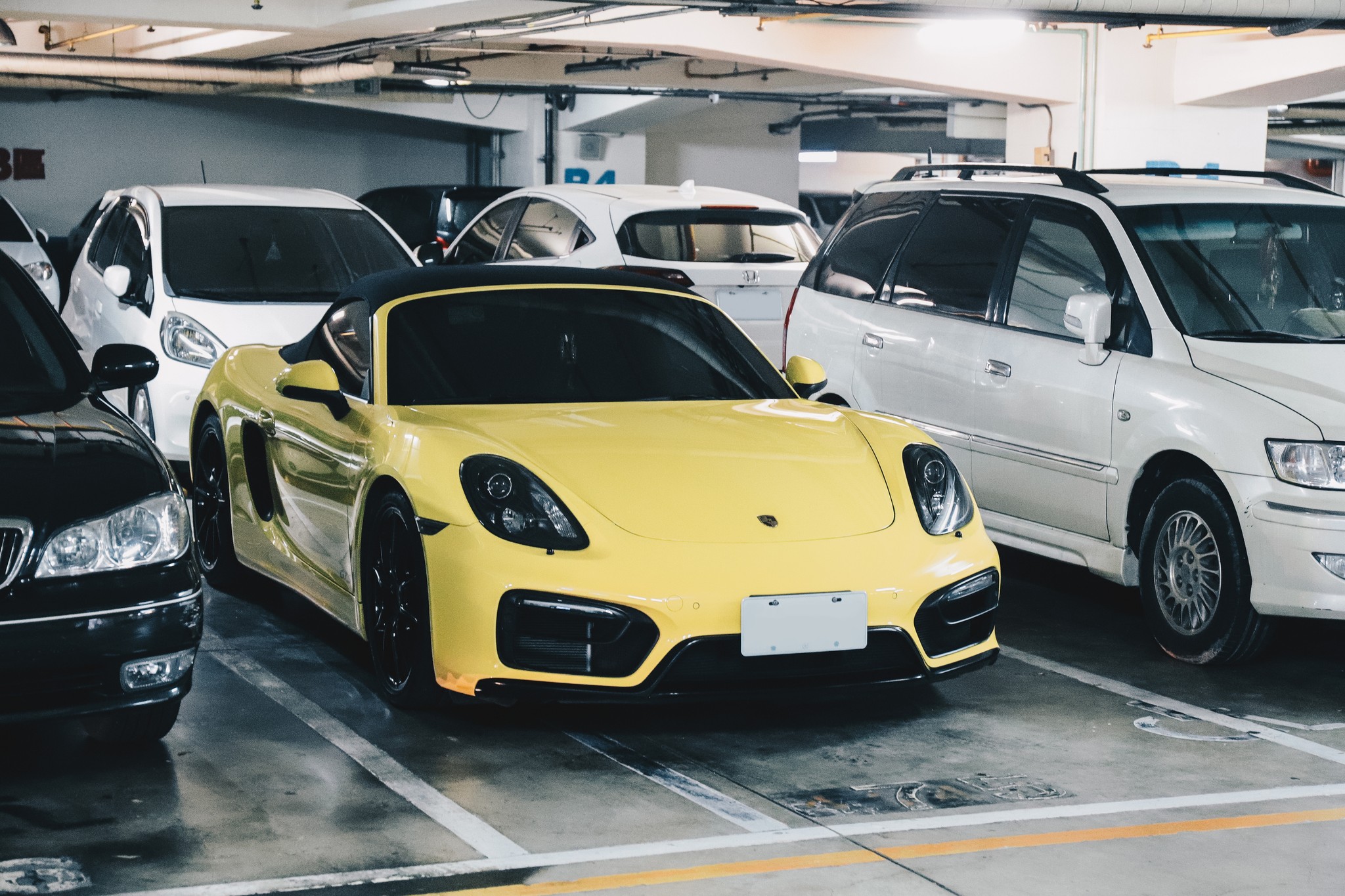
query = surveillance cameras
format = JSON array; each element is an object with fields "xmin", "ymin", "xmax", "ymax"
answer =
[{"xmin": 709, "ymin": 94, "xmax": 720, "ymax": 104}]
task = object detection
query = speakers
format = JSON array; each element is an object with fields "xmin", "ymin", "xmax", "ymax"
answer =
[{"xmin": 578, "ymin": 134, "xmax": 608, "ymax": 160}]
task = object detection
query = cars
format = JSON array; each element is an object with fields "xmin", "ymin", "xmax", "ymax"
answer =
[
  {"xmin": 0, "ymin": 193, "xmax": 61, "ymax": 313},
  {"xmin": 0, "ymin": 248, "xmax": 201, "ymax": 747},
  {"xmin": 356, "ymin": 183, "xmax": 526, "ymax": 271},
  {"xmin": 417, "ymin": 180, "xmax": 831, "ymax": 385},
  {"xmin": 63, "ymin": 184, "xmax": 426, "ymax": 465}
]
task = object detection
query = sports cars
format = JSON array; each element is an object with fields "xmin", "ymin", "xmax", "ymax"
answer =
[{"xmin": 188, "ymin": 265, "xmax": 1004, "ymax": 721}]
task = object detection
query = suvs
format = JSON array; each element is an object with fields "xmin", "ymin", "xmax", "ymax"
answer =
[{"xmin": 783, "ymin": 164, "xmax": 1344, "ymax": 673}]
